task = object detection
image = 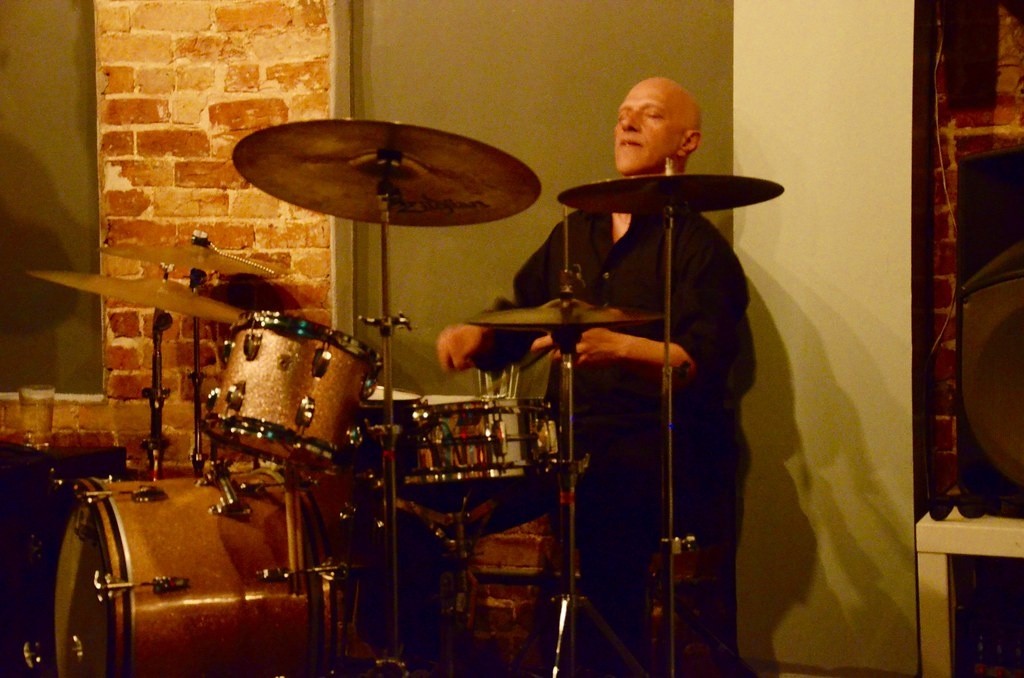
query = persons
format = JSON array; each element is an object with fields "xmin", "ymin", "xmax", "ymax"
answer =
[{"xmin": 436, "ymin": 75, "xmax": 757, "ymax": 677}]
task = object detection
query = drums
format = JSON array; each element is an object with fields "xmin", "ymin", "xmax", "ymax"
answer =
[
  {"xmin": 401, "ymin": 394, "xmax": 564, "ymax": 499},
  {"xmin": 41, "ymin": 462, "xmax": 341, "ymax": 678},
  {"xmin": 460, "ymin": 510, "xmax": 587, "ymax": 678},
  {"xmin": 200, "ymin": 310, "xmax": 380, "ymax": 475}
]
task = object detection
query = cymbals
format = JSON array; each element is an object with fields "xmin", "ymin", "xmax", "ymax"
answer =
[
  {"xmin": 95, "ymin": 240, "xmax": 281, "ymax": 279},
  {"xmin": 232, "ymin": 117, "xmax": 544, "ymax": 227},
  {"xmin": 556, "ymin": 173, "xmax": 785, "ymax": 216},
  {"xmin": 26, "ymin": 270, "xmax": 245, "ymax": 322},
  {"xmin": 365, "ymin": 384, "xmax": 421, "ymax": 402},
  {"xmin": 465, "ymin": 297, "xmax": 667, "ymax": 334}
]
